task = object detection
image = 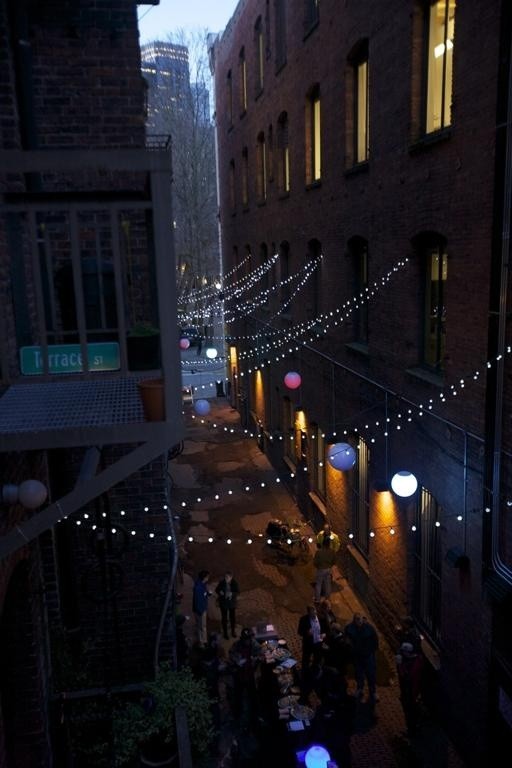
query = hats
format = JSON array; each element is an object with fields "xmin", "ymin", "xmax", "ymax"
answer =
[
  {"xmin": 400, "ymin": 615, "xmax": 414, "ymax": 625},
  {"xmin": 399, "ymin": 642, "xmax": 414, "ymax": 652}
]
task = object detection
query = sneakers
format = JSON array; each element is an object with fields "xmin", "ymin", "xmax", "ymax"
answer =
[
  {"xmin": 312, "ymin": 595, "xmax": 331, "ymax": 604},
  {"xmin": 369, "ymin": 691, "xmax": 380, "ymax": 702},
  {"xmin": 352, "ymin": 688, "xmax": 365, "ymax": 697}
]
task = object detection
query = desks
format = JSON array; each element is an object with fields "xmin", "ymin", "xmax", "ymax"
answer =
[{"xmin": 257, "ymin": 638, "xmax": 312, "ymax": 768}]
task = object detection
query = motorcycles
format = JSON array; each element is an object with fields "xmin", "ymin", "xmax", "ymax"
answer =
[{"xmin": 264, "ymin": 517, "xmax": 316, "ymax": 564}]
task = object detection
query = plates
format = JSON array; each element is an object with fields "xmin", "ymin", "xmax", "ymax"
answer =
[
  {"xmin": 290, "ymin": 706, "xmax": 313, "ymax": 721},
  {"xmin": 278, "ymin": 696, "xmax": 302, "ymax": 708}
]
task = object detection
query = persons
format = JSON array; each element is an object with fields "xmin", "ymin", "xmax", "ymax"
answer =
[{"xmin": 192, "ymin": 523, "xmax": 426, "ymax": 737}]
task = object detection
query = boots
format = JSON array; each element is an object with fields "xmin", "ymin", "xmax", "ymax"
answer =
[
  {"xmin": 222, "ymin": 624, "xmax": 229, "ymax": 640},
  {"xmin": 231, "ymin": 623, "xmax": 237, "ymax": 638}
]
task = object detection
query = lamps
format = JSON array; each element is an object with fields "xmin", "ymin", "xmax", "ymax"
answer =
[
  {"xmin": 391, "ymin": 470, "xmax": 418, "ymax": 498},
  {"xmin": 327, "ymin": 442, "xmax": 356, "ymax": 472},
  {"xmin": 206, "ymin": 348, "xmax": 218, "ymax": 358},
  {"xmin": 283, "ymin": 371, "xmax": 301, "ymax": 389},
  {"xmin": 193, "ymin": 400, "xmax": 211, "ymax": 417},
  {"xmin": 1, "ymin": 478, "xmax": 47, "ymax": 510},
  {"xmin": 304, "ymin": 745, "xmax": 331, "ymax": 768},
  {"xmin": 179, "ymin": 338, "xmax": 190, "ymax": 350}
]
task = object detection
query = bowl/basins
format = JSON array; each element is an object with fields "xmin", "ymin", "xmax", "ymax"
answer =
[
  {"xmin": 277, "ymin": 638, "xmax": 288, "ymax": 646},
  {"xmin": 266, "ymin": 639, "xmax": 277, "ymax": 650},
  {"xmin": 272, "ymin": 649, "xmax": 292, "ymax": 663}
]
